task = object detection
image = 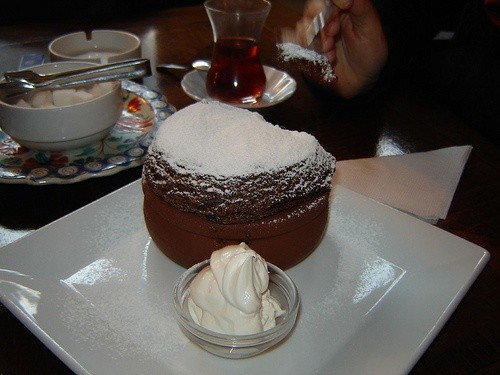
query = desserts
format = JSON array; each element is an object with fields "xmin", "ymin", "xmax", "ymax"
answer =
[
  {"xmin": 141, "ymin": 98, "xmax": 337, "ymax": 274},
  {"xmin": 172, "ymin": 241, "xmax": 300, "ymax": 359}
]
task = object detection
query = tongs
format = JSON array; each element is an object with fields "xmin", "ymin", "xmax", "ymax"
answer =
[{"xmin": 0, "ymin": 58, "xmax": 153, "ymax": 105}]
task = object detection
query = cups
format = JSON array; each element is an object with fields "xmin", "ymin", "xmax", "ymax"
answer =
[{"xmin": 204, "ymin": 0, "xmax": 271, "ymax": 103}]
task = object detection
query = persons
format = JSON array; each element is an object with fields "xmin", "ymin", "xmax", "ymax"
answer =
[{"xmin": 295, "ymin": 1, "xmax": 500, "ymax": 104}]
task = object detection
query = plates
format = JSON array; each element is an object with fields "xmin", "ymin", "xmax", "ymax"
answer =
[
  {"xmin": 0, "ymin": 78, "xmax": 176, "ymax": 184},
  {"xmin": 181, "ymin": 65, "xmax": 296, "ymax": 108},
  {"xmin": 0, "ymin": 177, "xmax": 491, "ymax": 375}
]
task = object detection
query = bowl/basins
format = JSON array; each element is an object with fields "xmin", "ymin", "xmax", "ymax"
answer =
[
  {"xmin": 0, "ymin": 61, "xmax": 121, "ymax": 152},
  {"xmin": 174, "ymin": 259, "xmax": 300, "ymax": 359}
]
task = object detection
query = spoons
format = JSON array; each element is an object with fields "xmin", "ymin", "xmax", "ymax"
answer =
[{"xmin": 156, "ymin": 60, "xmax": 211, "ymax": 71}]
